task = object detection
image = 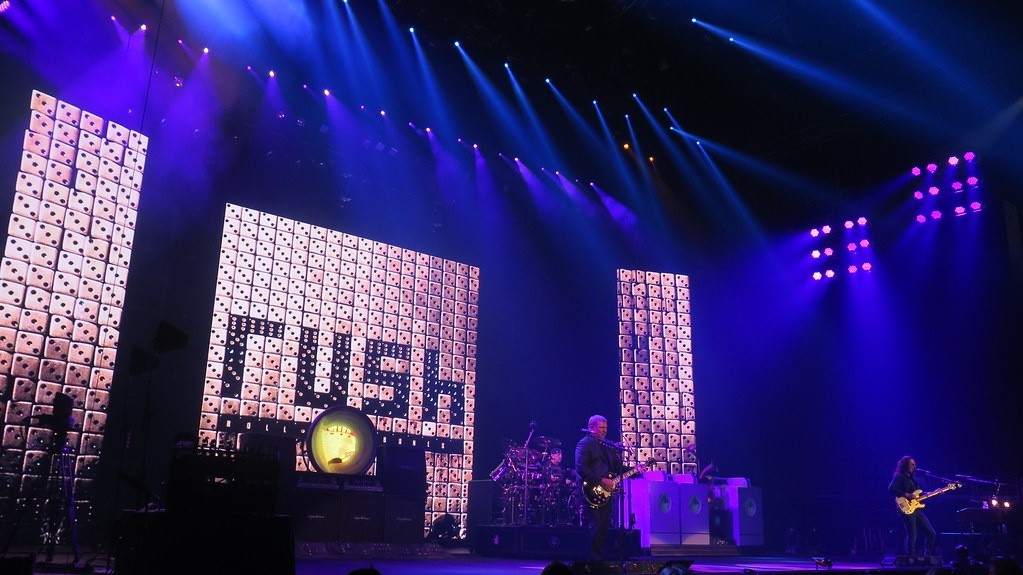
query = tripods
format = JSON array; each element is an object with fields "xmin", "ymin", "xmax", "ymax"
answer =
[{"xmin": 0, "ymin": 435, "xmax": 83, "ymax": 570}]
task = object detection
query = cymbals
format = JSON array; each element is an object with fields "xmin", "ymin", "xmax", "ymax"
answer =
[
  {"xmin": 534, "ymin": 435, "xmax": 562, "ymax": 447},
  {"xmin": 509, "ymin": 447, "xmax": 542, "ymax": 470}
]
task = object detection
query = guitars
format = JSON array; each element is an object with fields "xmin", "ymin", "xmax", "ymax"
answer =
[
  {"xmin": 582, "ymin": 457, "xmax": 658, "ymax": 510},
  {"xmin": 896, "ymin": 481, "xmax": 963, "ymax": 515}
]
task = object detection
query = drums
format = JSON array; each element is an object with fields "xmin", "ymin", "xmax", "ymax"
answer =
[
  {"xmin": 489, "ymin": 459, "xmax": 519, "ymax": 487},
  {"xmin": 566, "ymin": 487, "xmax": 580, "ymax": 521}
]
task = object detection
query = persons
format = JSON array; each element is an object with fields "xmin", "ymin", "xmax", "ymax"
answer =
[
  {"xmin": 545, "ymin": 448, "xmax": 577, "ymax": 487},
  {"xmin": 575, "ymin": 415, "xmax": 648, "ymax": 560},
  {"xmin": 888, "ymin": 456, "xmax": 938, "ymax": 565}
]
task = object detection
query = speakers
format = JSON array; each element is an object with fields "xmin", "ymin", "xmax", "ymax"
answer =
[
  {"xmin": 569, "ymin": 560, "xmax": 667, "ymax": 575},
  {"xmin": 467, "ymin": 479, "xmax": 502, "ymax": 552},
  {"xmin": 240, "ymin": 432, "xmax": 426, "ymax": 545}
]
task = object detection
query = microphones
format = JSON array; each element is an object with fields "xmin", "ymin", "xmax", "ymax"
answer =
[
  {"xmin": 915, "ymin": 467, "xmax": 931, "ymax": 473},
  {"xmin": 956, "ymin": 475, "xmax": 971, "ymax": 478}
]
